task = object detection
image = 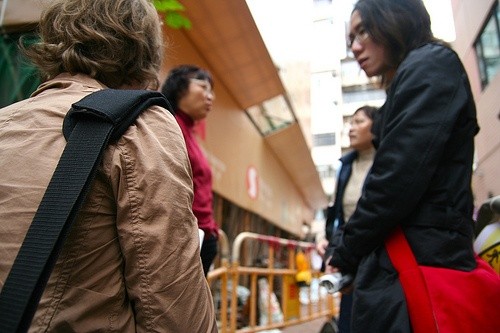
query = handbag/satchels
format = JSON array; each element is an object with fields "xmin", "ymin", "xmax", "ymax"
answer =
[{"xmin": 398, "ymin": 252, "xmax": 500, "ymax": 333}]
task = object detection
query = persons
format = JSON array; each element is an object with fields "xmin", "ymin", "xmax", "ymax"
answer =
[
  {"xmin": 314, "ymin": 105, "xmax": 380, "ymax": 273},
  {"xmin": 319, "ymin": 0, "xmax": 481, "ymax": 333},
  {"xmin": 0, "ymin": 0, "xmax": 226, "ymax": 333},
  {"xmin": 162, "ymin": 63, "xmax": 222, "ymax": 279}
]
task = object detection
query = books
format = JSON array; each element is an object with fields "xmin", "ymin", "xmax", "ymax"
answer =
[{"xmin": 318, "ymin": 269, "xmax": 353, "ymax": 294}]
